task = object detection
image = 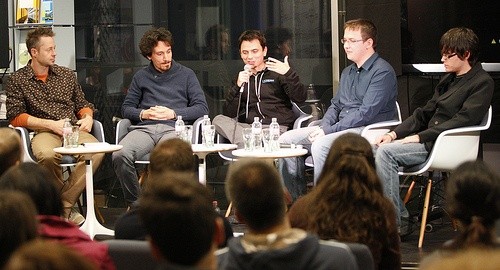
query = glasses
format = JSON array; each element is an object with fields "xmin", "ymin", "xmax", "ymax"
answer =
[
  {"xmin": 340, "ymin": 38, "xmax": 365, "ymax": 44},
  {"xmin": 443, "ymin": 52, "xmax": 457, "ymax": 59}
]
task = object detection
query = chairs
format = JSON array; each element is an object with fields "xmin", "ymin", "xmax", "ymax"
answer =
[
  {"xmin": 398, "ymin": 106, "xmax": 492, "ymax": 247},
  {"xmin": 217, "ymin": 101, "xmax": 313, "ymax": 217},
  {"xmin": 306, "ymin": 101, "xmax": 403, "ymax": 168},
  {"xmin": 9, "ymin": 120, "xmax": 106, "ymax": 224},
  {"xmin": 116, "ymin": 117, "xmax": 210, "ymax": 216}
]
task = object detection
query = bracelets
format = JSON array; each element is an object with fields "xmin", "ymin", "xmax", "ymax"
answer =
[{"xmin": 384, "ymin": 133, "xmax": 395, "ymax": 141}]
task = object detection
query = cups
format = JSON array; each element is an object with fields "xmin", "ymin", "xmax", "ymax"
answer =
[
  {"xmin": 72, "ymin": 126, "xmax": 79, "ymax": 148},
  {"xmin": 182, "ymin": 125, "xmax": 193, "ymax": 145},
  {"xmin": 204, "ymin": 125, "xmax": 215, "ymax": 147},
  {"xmin": 262, "ymin": 129, "xmax": 273, "ymax": 152},
  {"xmin": 242, "ymin": 128, "xmax": 254, "ymax": 150}
]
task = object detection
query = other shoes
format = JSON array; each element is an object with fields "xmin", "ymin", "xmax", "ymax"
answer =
[{"xmin": 64, "ymin": 206, "xmax": 85, "ymax": 226}]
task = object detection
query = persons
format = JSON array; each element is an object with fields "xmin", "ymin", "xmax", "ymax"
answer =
[
  {"xmin": 312, "ymin": 27, "xmax": 353, "ymax": 115},
  {"xmin": 414, "ymin": 31, "xmax": 449, "ymax": 105},
  {"xmin": 113, "ymin": 138, "xmax": 234, "ymax": 251},
  {"xmin": 0, "ymin": 127, "xmax": 116, "ymax": 270},
  {"xmin": 138, "ymin": 173, "xmax": 226, "ymax": 269},
  {"xmin": 288, "ymin": 132, "xmax": 401, "ymax": 270},
  {"xmin": 211, "ymin": 29, "xmax": 307, "ymax": 212},
  {"xmin": 419, "ymin": 160, "xmax": 500, "ymax": 270},
  {"xmin": 110, "ymin": 27, "xmax": 208, "ymax": 209},
  {"xmin": 264, "ymin": 28, "xmax": 293, "ymax": 67},
  {"xmin": 181, "ymin": 25, "xmax": 232, "ymax": 96},
  {"xmin": 80, "ymin": 59, "xmax": 107, "ymax": 105},
  {"xmin": 216, "ymin": 158, "xmax": 358, "ymax": 269},
  {"xmin": 6, "ymin": 27, "xmax": 104, "ymax": 226},
  {"xmin": 277, "ymin": 18, "xmax": 398, "ymax": 209},
  {"xmin": 368, "ymin": 26, "xmax": 495, "ymax": 246}
]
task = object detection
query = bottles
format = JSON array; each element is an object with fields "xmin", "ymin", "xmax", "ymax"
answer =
[
  {"xmin": 175, "ymin": 116, "xmax": 184, "ymax": 139},
  {"xmin": 201, "ymin": 115, "xmax": 211, "ymax": 145},
  {"xmin": 308, "ymin": 84, "xmax": 314, "ymax": 99},
  {"xmin": 270, "ymin": 118, "xmax": 280, "ymax": 151},
  {"xmin": 63, "ymin": 118, "xmax": 72, "ymax": 148},
  {"xmin": 252, "ymin": 117, "xmax": 262, "ymax": 150}
]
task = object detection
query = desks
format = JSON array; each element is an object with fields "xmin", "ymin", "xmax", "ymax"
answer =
[
  {"xmin": 403, "ymin": 64, "xmax": 500, "ymax": 117},
  {"xmin": 52, "ymin": 143, "xmax": 123, "ymax": 241},
  {"xmin": 232, "ymin": 148, "xmax": 309, "ymax": 168},
  {"xmin": 187, "ymin": 144, "xmax": 238, "ymax": 189}
]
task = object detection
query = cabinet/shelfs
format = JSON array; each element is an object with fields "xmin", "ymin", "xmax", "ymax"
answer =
[
  {"xmin": 14, "ymin": 0, "xmax": 78, "ymax": 82},
  {"xmin": 79, "ymin": 1, "xmax": 157, "ymax": 69}
]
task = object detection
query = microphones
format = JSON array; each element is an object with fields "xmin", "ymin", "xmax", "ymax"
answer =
[{"xmin": 239, "ymin": 64, "xmax": 251, "ymax": 92}]
